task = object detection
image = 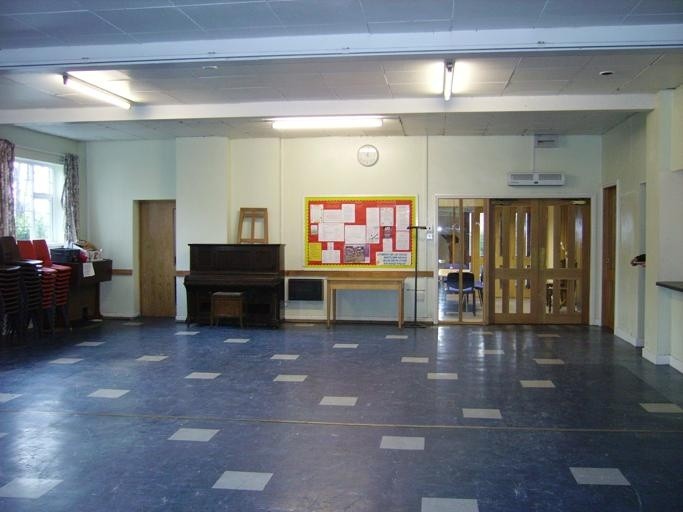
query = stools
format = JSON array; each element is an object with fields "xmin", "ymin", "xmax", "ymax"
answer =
[{"xmin": 211, "ymin": 291, "xmax": 247, "ymax": 327}]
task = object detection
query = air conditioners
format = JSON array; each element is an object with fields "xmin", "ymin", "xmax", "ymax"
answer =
[{"xmin": 507, "ymin": 172, "xmax": 565, "ymax": 187}]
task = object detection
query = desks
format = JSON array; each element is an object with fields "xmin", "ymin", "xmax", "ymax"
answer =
[
  {"xmin": 439, "ymin": 269, "xmax": 469, "ymax": 295},
  {"xmin": 325, "ymin": 276, "xmax": 404, "ymax": 328}
]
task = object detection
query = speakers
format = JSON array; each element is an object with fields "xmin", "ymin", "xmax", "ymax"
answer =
[{"xmin": 288, "ymin": 279, "xmax": 323, "ymax": 301}]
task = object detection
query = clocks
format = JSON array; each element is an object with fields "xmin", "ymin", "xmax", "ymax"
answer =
[{"xmin": 355, "ymin": 144, "xmax": 379, "ymax": 170}]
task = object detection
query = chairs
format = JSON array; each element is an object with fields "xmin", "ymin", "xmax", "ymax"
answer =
[
  {"xmin": 447, "ymin": 264, "xmax": 486, "ymax": 320},
  {"xmin": 0, "ymin": 236, "xmax": 72, "ymax": 349}
]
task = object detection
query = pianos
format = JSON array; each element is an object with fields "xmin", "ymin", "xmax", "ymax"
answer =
[{"xmin": 183, "ymin": 244, "xmax": 286, "ymax": 330}]
